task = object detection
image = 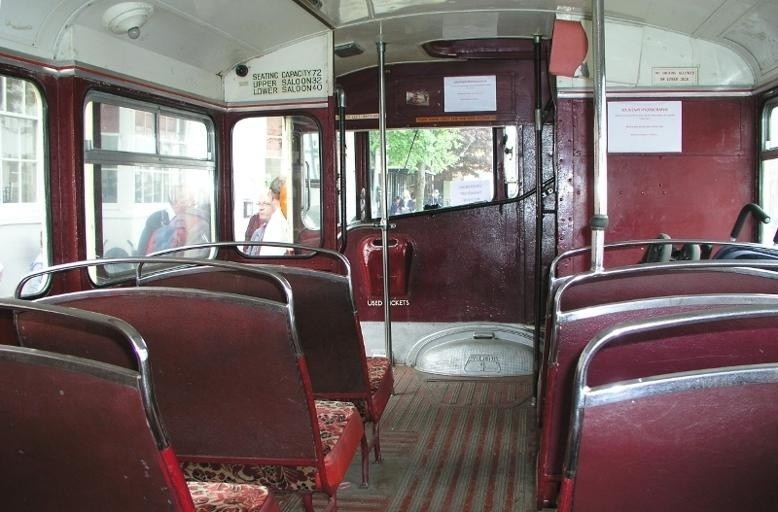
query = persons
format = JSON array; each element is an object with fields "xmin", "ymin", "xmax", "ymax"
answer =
[
  {"xmin": 259, "ymin": 174, "xmax": 291, "ymax": 257},
  {"xmin": 146, "ymin": 182, "xmax": 209, "ymax": 269},
  {"xmin": 389, "ymin": 190, "xmax": 441, "ymax": 215},
  {"xmin": 245, "ymin": 187, "xmax": 276, "ymax": 257}
]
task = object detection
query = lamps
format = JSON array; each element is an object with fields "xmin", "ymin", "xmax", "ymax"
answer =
[{"xmin": 101, "ymin": 2, "xmax": 154, "ymax": 41}]
